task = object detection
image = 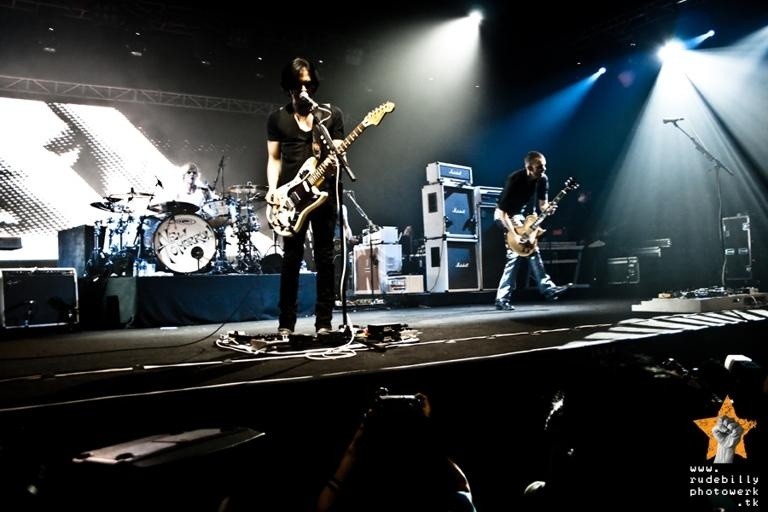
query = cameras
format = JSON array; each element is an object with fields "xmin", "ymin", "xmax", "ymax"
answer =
[{"xmin": 378, "ymin": 395, "xmax": 419, "ymax": 411}]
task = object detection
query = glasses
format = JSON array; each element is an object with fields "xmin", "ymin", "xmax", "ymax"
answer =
[{"xmin": 186, "ymin": 171, "xmax": 196, "ymax": 175}]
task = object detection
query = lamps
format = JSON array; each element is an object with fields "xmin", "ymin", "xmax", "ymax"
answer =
[
  {"xmin": 41, "ymin": 25, "xmax": 58, "ymax": 55},
  {"xmin": 129, "ymin": 30, "xmax": 143, "ymax": 57}
]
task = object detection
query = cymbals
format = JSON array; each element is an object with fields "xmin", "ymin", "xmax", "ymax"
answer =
[
  {"xmin": 106, "ymin": 187, "xmax": 153, "ymax": 198},
  {"xmin": 90, "ymin": 201, "xmax": 134, "ymax": 213},
  {"xmin": 228, "ymin": 182, "xmax": 269, "ymax": 196}
]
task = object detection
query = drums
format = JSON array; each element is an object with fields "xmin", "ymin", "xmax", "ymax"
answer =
[
  {"xmin": 138, "ymin": 210, "xmax": 217, "ymax": 274},
  {"xmin": 147, "ymin": 201, "xmax": 201, "ymax": 213},
  {"xmin": 239, "ymin": 212, "xmax": 262, "ymax": 232},
  {"xmin": 203, "ymin": 200, "xmax": 237, "ymax": 227}
]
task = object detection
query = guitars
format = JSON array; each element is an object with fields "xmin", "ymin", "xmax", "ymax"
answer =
[
  {"xmin": 266, "ymin": 101, "xmax": 396, "ymax": 237},
  {"xmin": 505, "ymin": 177, "xmax": 579, "ymax": 257}
]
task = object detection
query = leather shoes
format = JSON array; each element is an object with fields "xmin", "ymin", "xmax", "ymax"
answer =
[
  {"xmin": 545, "ymin": 281, "xmax": 574, "ymax": 300},
  {"xmin": 496, "ymin": 302, "xmax": 515, "ymax": 311}
]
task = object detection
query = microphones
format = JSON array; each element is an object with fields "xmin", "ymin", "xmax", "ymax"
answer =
[
  {"xmin": 300, "ymin": 92, "xmax": 318, "ymax": 109},
  {"xmin": 204, "ymin": 180, "xmax": 213, "ymax": 191},
  {"xmin": 343, "ymin": 189, "xmax": 352, "ymax": 194},
  {"xmin": 662, "ymin": 117, "xmax": 683, "ymax": 124}
]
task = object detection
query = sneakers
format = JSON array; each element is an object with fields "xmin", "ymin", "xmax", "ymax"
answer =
[
  {"xmin": 278, "ymin": 327, "xmax": 292, "ymax": 341},
  {"xmin": 315, "ymin": 328, "xmax": 331, "ymax": 336}
]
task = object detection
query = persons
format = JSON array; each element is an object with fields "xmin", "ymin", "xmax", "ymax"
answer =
[
  {"xmin": 493, "ymin": 150, "xmax": 575, "ymax": 312},
  {"xmin": 332, "ymin": 203, "xmax": 356, "ymax": 308},
  {"xmin": 263, "ymin": 55, "xmax": 349, "ymax": 341},
  {"xmin": 171, "ymin": 161, "xmax": 211, "ymax": 209},
  {"xmin": 520, "ymin": 382, "xmax": 637, "ymax": 511},
  {"xmin": 399, "ymin": 224, "xmax": 419, "ymax": 256},
  {"xmin": 315, "ymin": 387, "xmax": 479, "ymax": 511}
]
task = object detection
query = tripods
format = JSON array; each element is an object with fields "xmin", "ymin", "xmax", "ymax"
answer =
[{"xmin": 338, "ymin": 164, "xmax": 386, "ymax": 353}]
